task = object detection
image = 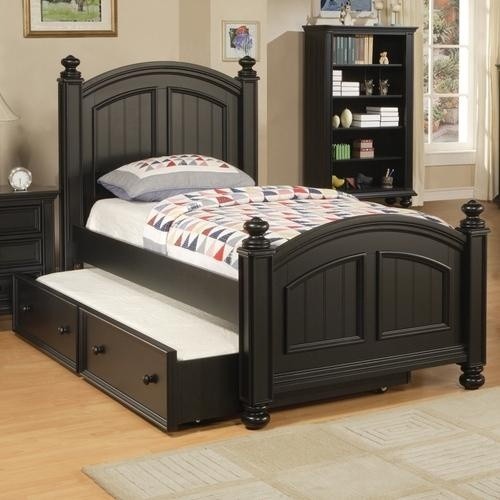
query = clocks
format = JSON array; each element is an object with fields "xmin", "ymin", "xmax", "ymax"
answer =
[{"xmin": 9, "ymin": 166, "xmax": 34, "ymax": 192}]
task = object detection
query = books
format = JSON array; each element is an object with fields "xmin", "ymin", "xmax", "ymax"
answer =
[{"xmin": 331, "ymin": 34, "xmax": 401, "ymax": 160}]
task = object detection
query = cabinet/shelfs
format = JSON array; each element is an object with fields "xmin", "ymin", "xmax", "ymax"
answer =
[
  {"xmin": 302, "ymin": 25, "xmax": 419, "ymax": 210},
  {"xmin": 10, "ymin": 272, "xmax": 239, "ymax": 431}
]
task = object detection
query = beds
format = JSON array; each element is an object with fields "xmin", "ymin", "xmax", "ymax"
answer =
[{"xmin": 56, "ymin": 53, "xmax": 492, "ymax": 431}]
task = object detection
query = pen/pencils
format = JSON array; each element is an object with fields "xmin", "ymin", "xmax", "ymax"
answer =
[{"xmin": 386, "ymin": 168, "xmax": 395, "ymax": 177}]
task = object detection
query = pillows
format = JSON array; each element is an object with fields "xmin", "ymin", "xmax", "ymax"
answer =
[{"xmin": 93, "ymin": 153, "xmax": 256, "ymax": 203}]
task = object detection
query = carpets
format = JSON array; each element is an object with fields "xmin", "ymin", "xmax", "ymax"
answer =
[{"xmin": 82, "ymin": 385, "xmax": 498, "ymax": 500}]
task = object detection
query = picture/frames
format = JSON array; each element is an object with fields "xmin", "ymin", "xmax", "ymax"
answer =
[
  {"xmin": 220, "ymin": 19, "xmax": 262, "ymax": 62},
  {"xmin": 22, "ymin": 0, "xmax": 118, "ymax": 38},
  {"xmin": 311, "ymin": 0, "xmax": 378, "ymax": 19}
]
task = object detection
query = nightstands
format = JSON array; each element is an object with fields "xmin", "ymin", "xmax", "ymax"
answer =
[{"xmin": 0, "ymin": 183, "xmax": 60, "ymax": 315}]
